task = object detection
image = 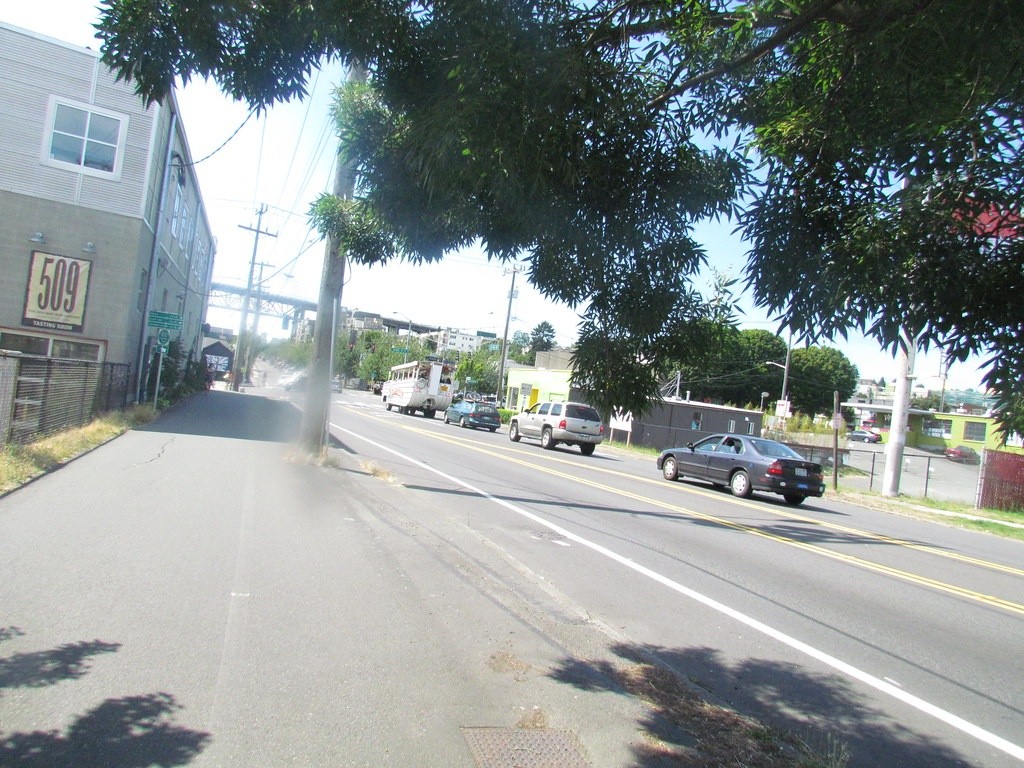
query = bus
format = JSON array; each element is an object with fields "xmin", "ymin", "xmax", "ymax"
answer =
[
  {"xmin": 386, "ymin": 360, "xmax": 455, "ymax": 418},
  {"xmin": 860, "ymin": 419, "xmax": 911, "ymax": 442}
]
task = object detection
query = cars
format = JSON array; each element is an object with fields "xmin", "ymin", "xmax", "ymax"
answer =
[
  {"xmin": 444, "ymin": 400, "xmax": 501, "ymax": 432},
  {"xmin": 845, "ymin": 430, "xmax": 882, "ymax": 443},
  {"xmin": 329, "ymin": 381, "xmax": 387, "ymax": 402},
  {"xmin": 655, "ymin": 431, "xmax": 825, "ymax": 506},
  {"xmin": 945, "ymin": 445, "xmax": 980, "ymax": 464}
]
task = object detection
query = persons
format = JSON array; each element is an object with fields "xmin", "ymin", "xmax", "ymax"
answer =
[{"xmin": 205, "ymin": 363, "xmax": 215, "ymax": 390}]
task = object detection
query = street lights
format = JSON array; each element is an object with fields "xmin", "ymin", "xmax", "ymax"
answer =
[
  {"xmin": 766, "ymin": 361, "xmax": 787, "ymax": 400},
  {"xmin": 392, "ymin": 311, "xmax": 412, "ymax": 365}
]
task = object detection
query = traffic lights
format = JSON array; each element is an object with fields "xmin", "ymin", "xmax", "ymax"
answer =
[{"xmin": 350, "ymin": 330, "xmax": 356, "ymax": 344}]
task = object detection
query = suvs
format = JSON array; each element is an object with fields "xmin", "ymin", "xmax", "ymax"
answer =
[{"xmin": 509, "ymin": 401, "xmax": 603, "ymax": 456}]
